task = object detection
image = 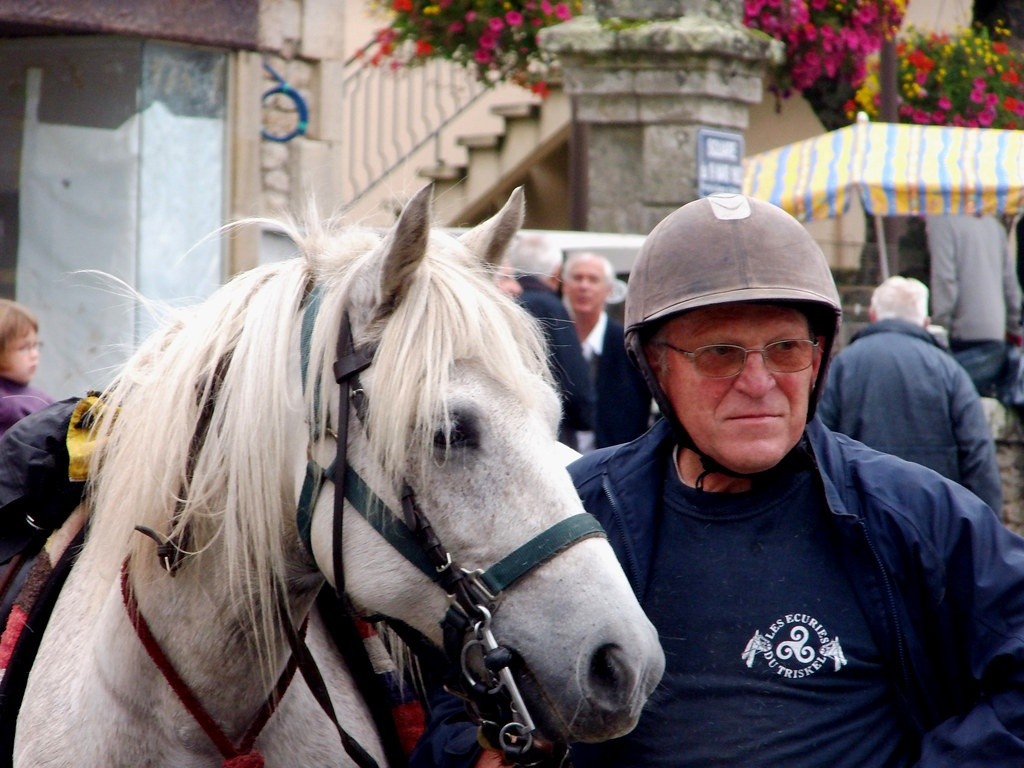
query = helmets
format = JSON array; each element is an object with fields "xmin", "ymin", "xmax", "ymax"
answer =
[{"xmin": 622, "ymin": 192, "xmax": 842, "ymax": 384}]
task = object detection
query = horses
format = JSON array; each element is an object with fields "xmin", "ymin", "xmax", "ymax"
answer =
[{"xmin": 0, "ymin": 181, "xmax": 668, "ymax": 768}]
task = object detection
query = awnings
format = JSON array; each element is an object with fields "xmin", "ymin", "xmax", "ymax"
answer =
[{"xmin": 745, "ymin": 112, "xmax": 1024, "ymax": 218}]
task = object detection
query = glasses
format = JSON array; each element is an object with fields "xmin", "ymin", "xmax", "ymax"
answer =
[
  {"xmin": 647, "ymin": 335, "xmax": 822, "ymax": 379},
  {"xmin": 8, "ymin": 341, "xmax": 44, "ymax": 355}
]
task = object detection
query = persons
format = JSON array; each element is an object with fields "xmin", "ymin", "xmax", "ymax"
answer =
[
  {"xmin": 0, "ymin": 299, "xmax": 59, "ymax": 433},
  {"xmin": 415, "ymin": 194, "xmax": 1023, "ymax": 768},
  {"xmin": 487, "ymin": 234, "xmax": 653, "ymax": 454},
  {"xmin": 926, "ymin": 218, "xmax": 1024, "ymax": 400},
  {"xmin": 816, "ymin": 276, "xmax": 1003, "ymax": 524}
]
